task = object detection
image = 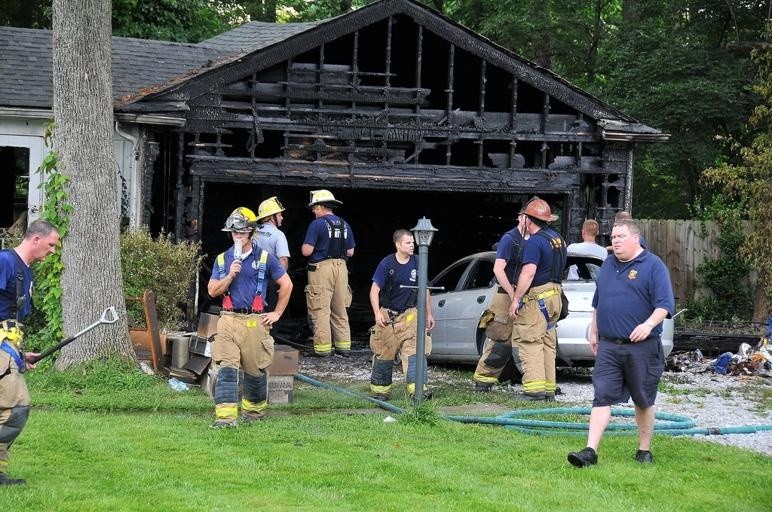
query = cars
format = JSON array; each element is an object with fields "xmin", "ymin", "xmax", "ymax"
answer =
[{"xmin": 430, "ymin": 242, "xmax": 679, "ymax": 372}]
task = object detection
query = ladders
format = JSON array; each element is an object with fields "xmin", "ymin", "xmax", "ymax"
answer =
[{"xmin": 123, "ymin": 289, "xmax": 162, "ymax": 372}]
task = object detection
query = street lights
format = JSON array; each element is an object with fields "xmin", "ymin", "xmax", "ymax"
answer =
[{"xmin": 406, "ymin": 212, "xmax": 436, "ymax": 405}]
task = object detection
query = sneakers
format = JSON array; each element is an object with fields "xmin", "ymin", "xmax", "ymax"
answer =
[
  {"xmin": 635, "ymin": 449, "xmax": 653, "ymax": 463},
  {"xmin": 369, "ymin": 390, "xmax": 433, "ymax": 406},
  {"xmin": 210, "ymin": 410, "xmax": 267, "ymax": 428},
  {"xmin": 475, "ymin": 382, "xmax": 561, "ymax": 402},
  {"xmin": 0, "ymin": 471, "xmax": 27, "ymax": 485},
  {"xmin": 299, "ymin": 345, "xmax": 351, "ymax": 357},
  {"xmin": 567, "ymin": 447, "xmax": 597, "ymax": 467}
]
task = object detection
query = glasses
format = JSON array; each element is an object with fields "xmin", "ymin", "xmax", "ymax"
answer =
[{"xmin": 224, "ymin": 216, "xmax": 256, "ymax": 230}]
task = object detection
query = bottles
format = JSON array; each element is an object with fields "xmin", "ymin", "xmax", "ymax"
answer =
[{"xmin": 234, "ymin": 239, "xmax": 243, "ymax": 262}]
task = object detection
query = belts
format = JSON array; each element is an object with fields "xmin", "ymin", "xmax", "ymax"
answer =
[
  {"xmin": 600, "ymin": 336, "xmax": 654, "ymax": 344},
  {"xmin": 0, "ymin": 321, "xmax": 24, "ymax": 331},
  {"xmin": 220, "ymin": 307, "xmax": 266, "ymax": 315}
]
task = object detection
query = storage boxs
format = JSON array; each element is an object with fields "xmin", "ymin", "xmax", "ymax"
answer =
[{"xmin": 184, "ymin": 312, "xmax": 299, "ymax": 405}]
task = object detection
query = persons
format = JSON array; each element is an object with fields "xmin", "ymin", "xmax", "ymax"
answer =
[
  {"xmin": 301, "ymin": 188, "xmax": 358, "ymax": 358},
  {"xmin": 368, "ymin": 228, "xmax": 436, "ymax": 403},
  {"xmin": 250, "ymin": 195, "xmax": 291, "ymax": 287},
  {"xmin": 0, "ymin": 219, "xmax": 61, "ymax": 488},
  {"xmin": 566, "ymin": 218, "xmax": 609, "ymax": 281},
  {"xmin": 471, "ymin": 200, "xmax": 563, "ymax": 397},
  {"xmin": 506, "ymin": 198, "xmax": 567, "ymax": 401},
  {"xmin": 603, "ymin": 209, "xmax": 648, "ymax": 254},
  {"xmin": 567, "ymin": 216, "xmax": 676, "ymax": 467},
  {"xmin": 207, "ymin": 205, "xmax": 295, "ymax": 429}
]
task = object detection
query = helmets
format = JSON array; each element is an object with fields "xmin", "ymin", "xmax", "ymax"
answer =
[
  {"xmin": 308, "ymin": 190, "xmax": 344, "ymax": 207},
  {"xmin": 523, "ymin": 197, "xmax": 559, "ymax": 222},
  {"xmin": 221, "ymin": 207, "xmax": 258, "ymax": 233},
  {"xmin": 256, "ymin": 196, "xmax": 286, "ymax": 221}
]
